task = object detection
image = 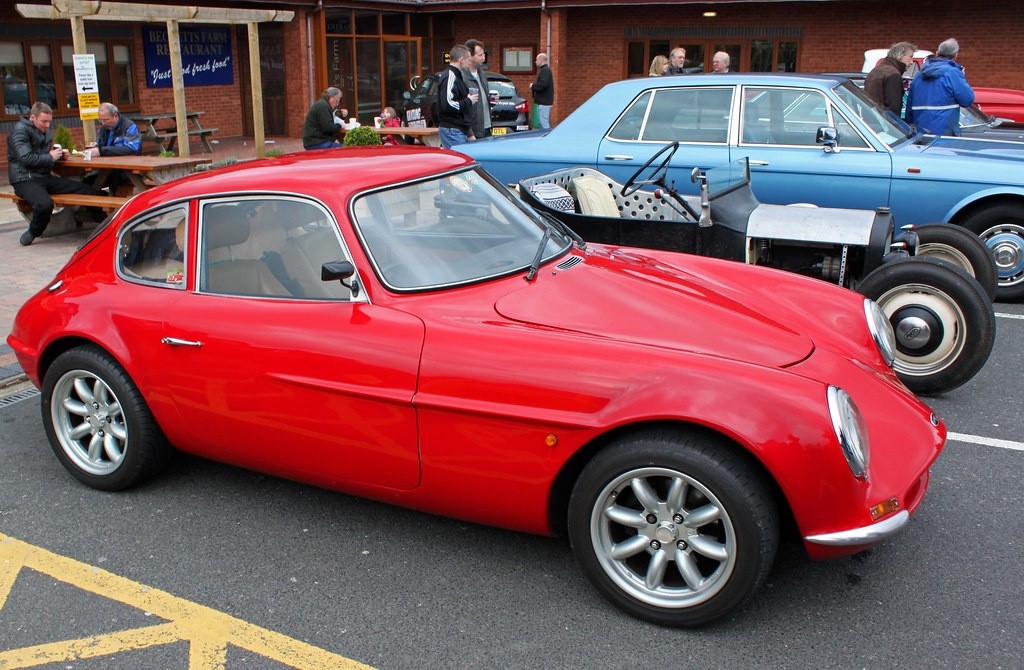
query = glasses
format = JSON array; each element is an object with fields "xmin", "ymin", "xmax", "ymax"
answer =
[{"xmin": 98, "ymin": 116, "xmax": 114, "ymax": 123}]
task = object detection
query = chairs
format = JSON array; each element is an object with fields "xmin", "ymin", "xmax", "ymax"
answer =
[
  {"xmin": 175, "ymin": 201, "xmax": 353, "ymax": 298},
  {"xmin": 742, "ymin": 103, "xmax": 777, "ymax": 145},
  {"xmin": 532, "ymin": 175, "xmax": 619, "ymax": 216}
]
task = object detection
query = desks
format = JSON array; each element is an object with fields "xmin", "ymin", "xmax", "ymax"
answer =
[
  {"xmin": 338, "ymin": 126, "xmax": 440, "ymax": 146},
  {"xmin": 53, "ymin": 155, "xmax": 212, "ymax": 191},
  {"xmin": 132, "ymin": 111, "xmax": 213, "ymax": 153}
]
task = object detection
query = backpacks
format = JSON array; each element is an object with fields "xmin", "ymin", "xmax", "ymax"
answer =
[{"xmin": 402, "ymin": 67, "xmax": 466, "ymax": 139}]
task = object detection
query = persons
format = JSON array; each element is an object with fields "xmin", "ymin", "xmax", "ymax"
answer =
[
  {"xmin": 864, "ymin": 41, "xmax": 916, "ymax": 124},
  {"xmin": 438, "ymin": 38, "xmax": 500, "ymax": 151},
  {"xmin": 698, "ymin": 50, "xmax": 737, "ymax": 110},
  {"xmin": 82, "ymin": 102, "xmax": 142, "ymax": 223},
  {"xmin": 905, "ymin": 37, "xmax": 975, "ymax": 137},
  {"xmin": 379, "ymin": 107, "xmax": 403, "ymax": 145},
  {"xmin": 647, "ymin": 47, "xmax": 687, "ymax": 122},
  {"xmin": 529, "ymin": 53, "xmax": 554, "ymax": 130},
  {"xmin": 303, "ymin": 86, "xmax": 348, "ymax": 149},
  {"xmin": 8, "ymin": 101, "xmax": 93, "ymax": 246}
]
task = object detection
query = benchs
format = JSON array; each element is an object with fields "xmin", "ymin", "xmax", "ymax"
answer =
[
  {"xmin": 620, "ymin": 104, "xmax": 676, "ymax": 139},
  {"xmin": 1, "ymin": 183, "xmax": 154, "ymax": 210},
  {"xmin": 137, "ymin": 126, "xmax": 219, "ymax": 141}
]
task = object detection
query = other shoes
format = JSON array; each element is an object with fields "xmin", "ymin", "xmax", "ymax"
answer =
[
  {"xmin": 86, "ymin": 206, "xmax": 107, "ymax": 223},
  {"xmin": 20, "ymin": 230, "xmax": 38, "ymax": 246}
]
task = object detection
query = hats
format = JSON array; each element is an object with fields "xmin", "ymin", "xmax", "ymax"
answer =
[{"xmin": 938, "ymin": 38, "xmax": 959, "ymax": 56}]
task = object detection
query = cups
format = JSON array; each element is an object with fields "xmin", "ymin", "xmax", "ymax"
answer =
[
  {"xmin": 469, "ymin": 88, "xmax": 478, "ymax": 96},
  {"xmin": 489, "ymin": 90, "xmax": 498, "ymax": 104},
  {"xmin": 349, "ymin": 118, "xmax": 356, "ymax": 128},
  {"xmin": 53, "ymin": 144, "xmax": 62, "ymax": 161},
  {"xmin": 374, "ymin": 117, "xmax": 381, "ymax": 128}
]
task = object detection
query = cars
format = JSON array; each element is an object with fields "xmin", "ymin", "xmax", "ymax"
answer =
[
  {"xmin": 400, "ymin": 71, "xmax": 532, "ymax": 147},
  {"xmin": 347, "ymin": 72, "xmax": 401, "ymax": 100},
  {"xmin": 388, "ymin": 64, "xmax": 415, "ymax": 84},
  {"xmin": 2, "ymin": 77, "xmax": 57, "ymax": 109},
  {"xmin": 723, "ymin": 72, "xmax": 1024, "ymax": 142},
  {"xmin": 433, "ymin": 72, "xmax": 1024, "ymax": 304},
  {"xmin": 365, "ymin": 140, "xmax": 998, "ymax": 396},
  {"xmin": 862, "ymin": 48, "xmax": 1024, "ymax": 125},
  {"xmin": 7, "ymin": 147, "xmax": 947, "ymax": 625}
]
